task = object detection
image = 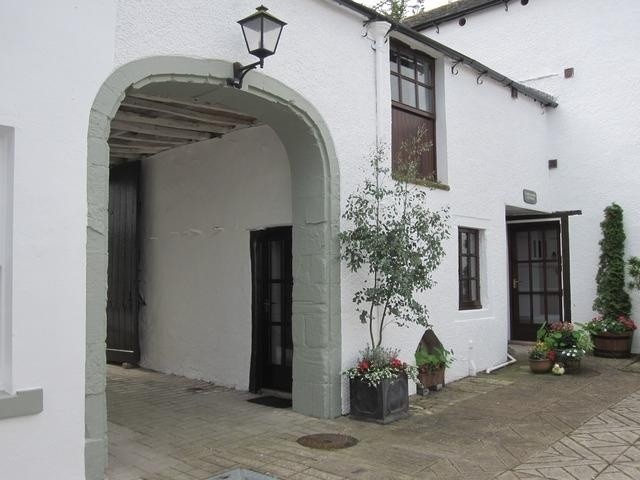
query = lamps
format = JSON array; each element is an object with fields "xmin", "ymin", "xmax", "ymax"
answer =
[{"xmin": 226, "ymin": 5, "xmax": 288, "ymax": 89}]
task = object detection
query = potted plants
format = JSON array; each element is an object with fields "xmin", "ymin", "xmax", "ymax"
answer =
[
  {"xmin": 338, "ymin": 121, "xmax": 455, "ymax": 425},
  {"xmin": 363, "ymin": 0, "xmax": 424, "ymax": 44},
  {"xmin": 415, "ymin": 343, "xmax": 455, "ymax": 393},
  {"xmin": 580, "ymin": 202, "xmax": 640, "ymax": 359}
]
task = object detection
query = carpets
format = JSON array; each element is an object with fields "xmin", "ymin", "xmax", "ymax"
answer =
[{"xmin": 247, "ymin": 395, "xmax": 291, "ymax": 409}]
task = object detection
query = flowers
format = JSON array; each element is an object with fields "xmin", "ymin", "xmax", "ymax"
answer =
[
  {"xmin": 529, "ymin": 320, "xmax": 595, "ymax": 375},
  {"xmin": 342, "ymin": 344, "xmax": 422, "ymax": 388},
  {"xmin": 588, "ymin": 317, "xmax": 638, "ymax": 333}
]
